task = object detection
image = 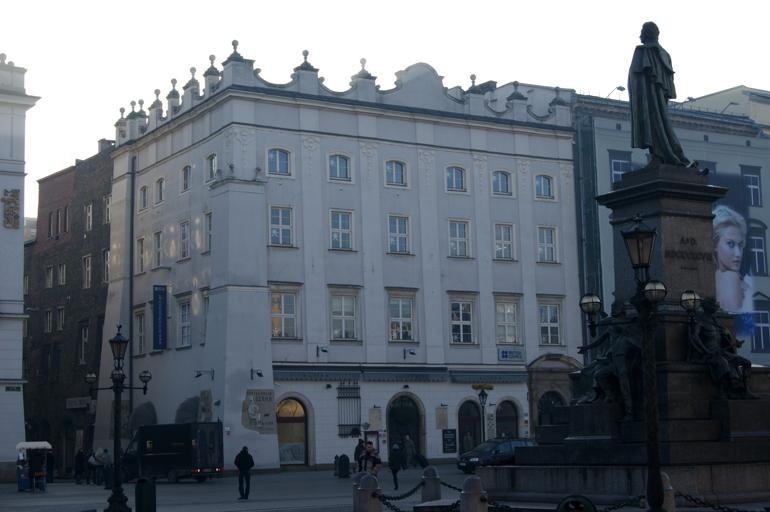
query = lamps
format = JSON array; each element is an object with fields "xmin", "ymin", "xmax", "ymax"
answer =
[
  {"xmin": 607, "ymin": 86, "xmax": 625, "ymax": 98},
  {"xmin": 317, "ymin": 345, "xmax": 329, "ymax": 357},
  {"xmin": 195, "ymin": 367, "xmax": 214, "ymax": 381},
  {"xmin": 721, "ymin": 102, "xmax": 739, "ymax": 113},
  {"xmin": 251, "ymin": 368, "xmax": 263, "ymax": 380},
  {"xmin": 404, "ymin": 348, "xmax": 416, "ymax": 359},
  {"xmin": 678, "ymin": 97, "xmax": 696, "ymax": 109}
]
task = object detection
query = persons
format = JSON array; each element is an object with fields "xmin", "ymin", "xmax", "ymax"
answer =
[
  {"xmin": 234, "ymin": 446, "xmax": 255, "ymax": 500},
  {"xmin": 628, "ymin": 21, "xmax": 690, "ymax": 166},
  {"xmin": 47, "ymin": 451, "xmax": 54, "ymax": 483},
  {"xmin": 29, "ymin": 453, "xmax": 43, "ymax": 488},
  {"xmin": 463, "ymin": 431, "xmax": 474, "ymax": 452},
  {"xmin": 74, "ymin": 448, "xmax": 108, "ymax": 485},
  {"xmin": 712, "ymin": 205, "xmax": 752, "ymax": 313},
  {"xmin": 354, "ymin": 434, "xmax": 416, "ymax": 491}
]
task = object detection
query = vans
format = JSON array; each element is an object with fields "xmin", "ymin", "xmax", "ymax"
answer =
[{"xmin": 456, "ymin": 437, "xmax": 540, "ymax": 475}]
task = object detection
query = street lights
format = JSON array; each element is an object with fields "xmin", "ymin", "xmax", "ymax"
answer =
[
  {"xmin": 478, "ymin": 388, "xmax": 488, "ymax": 444},
  {"xmin": 579, "ymin": 211, "xmax": 705, "ymax": 512},
  {"xmin": 85, "ymin": 323, "xmax": 152, "ymax": 512}
]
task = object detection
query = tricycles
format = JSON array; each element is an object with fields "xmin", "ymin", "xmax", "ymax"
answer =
[{"xmin": 16, "ymin": 440, "xmax": 52, "ymax": 497}]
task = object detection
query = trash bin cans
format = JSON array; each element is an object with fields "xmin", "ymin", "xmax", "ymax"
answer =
[{"xmin": 338, "ymin": 454, "xmax": 350, "ymax": 479}]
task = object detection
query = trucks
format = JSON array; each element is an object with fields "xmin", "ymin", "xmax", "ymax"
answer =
[{"xmin": 119, "ymin": 421, "xmax": 225, "ymax": 484}]
